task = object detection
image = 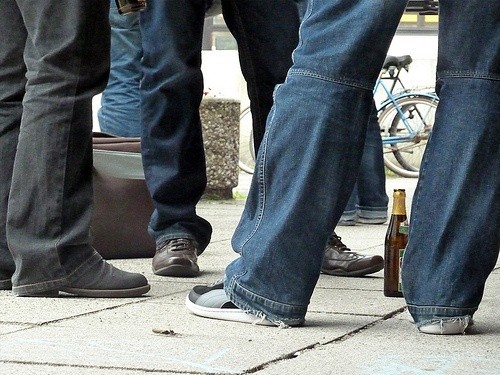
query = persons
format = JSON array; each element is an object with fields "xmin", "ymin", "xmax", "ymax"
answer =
[
  {"xmin": 297, "ymin": 0, "xmax": 388, "ymax": 225},
  {"xmin": 139, "ymin": 0, "xmax": 384, "ymax": 277},
  {"xmin": 0, "ymin": 0, "xmax": 151, "ymax": 297},
  {"xmin": 185, "ymin": 0, "xmax": 500, "ymax": 335},
  {"xmin": 98, "ymin": 0, "xmax": 143, "ymax": 137}
]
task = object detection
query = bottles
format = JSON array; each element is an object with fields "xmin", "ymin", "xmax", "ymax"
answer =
[{"xmin": 384, "ymin": 189, "xmax": 410, "ymax": 297}]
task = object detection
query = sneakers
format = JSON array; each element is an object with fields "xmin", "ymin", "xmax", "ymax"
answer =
[
  {"xmin": 406, "ymin": 306, "xmax": 466, "ymax": 334},
  {"xmin": 186, "ymin": 283, "xmax": 305, "ymax": 325}
]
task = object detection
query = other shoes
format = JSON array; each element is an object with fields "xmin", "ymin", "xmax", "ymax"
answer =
[
  {"xmin": 338, "ymin": 218, "xmax": 356, "ymax": 226},
  {"xmin": 357, "ymin": 214, "xmax": 386, "ymax": 225}
]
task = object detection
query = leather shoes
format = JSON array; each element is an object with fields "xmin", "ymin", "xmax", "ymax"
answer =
[
  {"xmin": 320, "ymin": 231, "xmax": 383, "ymax": 276},
  {"xmin": 15, "ymin": 259, "xmax": 150, "ymax": 297},
  {"xmin": 152, "ymin": 238, "xmax": 200, "ymax": 276}
]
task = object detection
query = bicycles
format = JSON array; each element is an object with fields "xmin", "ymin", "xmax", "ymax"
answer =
[{"xmin": 236, "ymin": 55, "xmax": 439, "ymax": 177}]
